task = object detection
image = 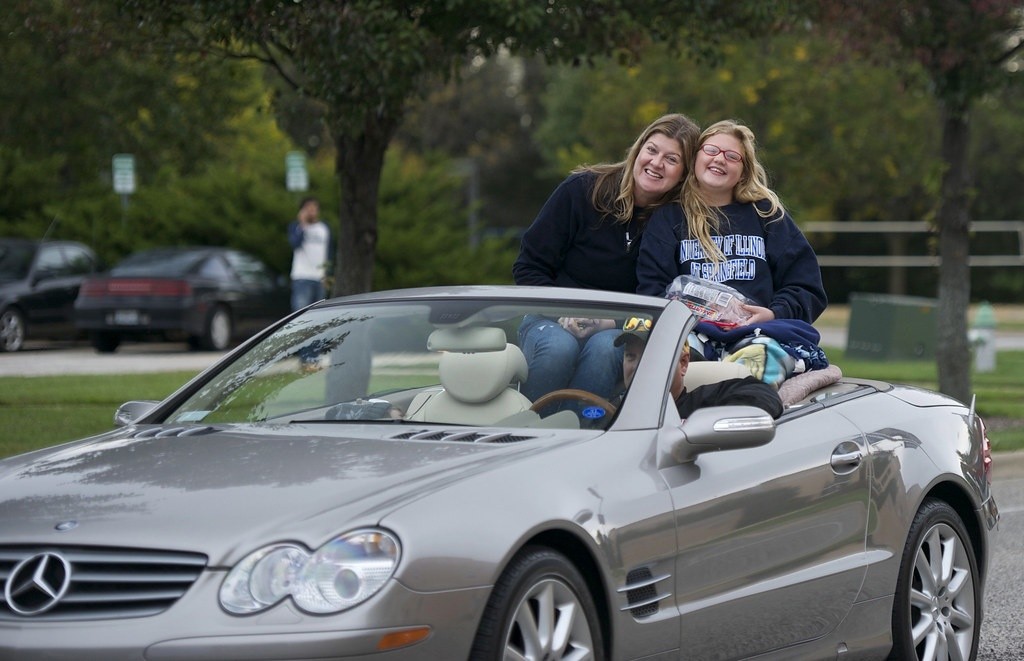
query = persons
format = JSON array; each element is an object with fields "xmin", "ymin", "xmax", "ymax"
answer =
[
  {"xmin": 513, "ymin": 113, "xmax": 701, "ymax": 429},
  {"xmin": 588, "ymin": 313, "xmax": 783, "ymax": 431},
  {"xmin": 286, "ymin": 196, "xmax": 332, "ymax": 312},
  {"xmin": 636, "ymin": 120, "xmax": 827, "ymax": 391}
]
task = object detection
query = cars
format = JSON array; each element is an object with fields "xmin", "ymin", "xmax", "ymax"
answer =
[
  {"xmin": 72, "ymin": 246, "xmax": 290, "ymax": 354},
  {"xmin": 0, "ymin": 283, "xmax": 1001, "ymax": 661},
  {"xmin": 1, "ymin": 237, "xmax": 106, "ymax": 352}
]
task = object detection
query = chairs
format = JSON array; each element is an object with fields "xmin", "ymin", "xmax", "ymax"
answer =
[
  {"xmin": 681, "ymin": 359, "xmax": 751, "ymax": 393},
  {"xmin": 403, "ymin": 341, "xmax": 534, "ymax": 427}
]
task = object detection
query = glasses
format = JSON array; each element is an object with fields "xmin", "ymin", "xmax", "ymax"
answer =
[{"xmin": 699, "ymin": 143, "xmax": 744, "ymax": 164}]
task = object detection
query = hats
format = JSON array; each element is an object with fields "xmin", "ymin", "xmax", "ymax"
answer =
[{"xmin": 611, "ymin": 312, "xmax": 690, "ymax": 356}]
